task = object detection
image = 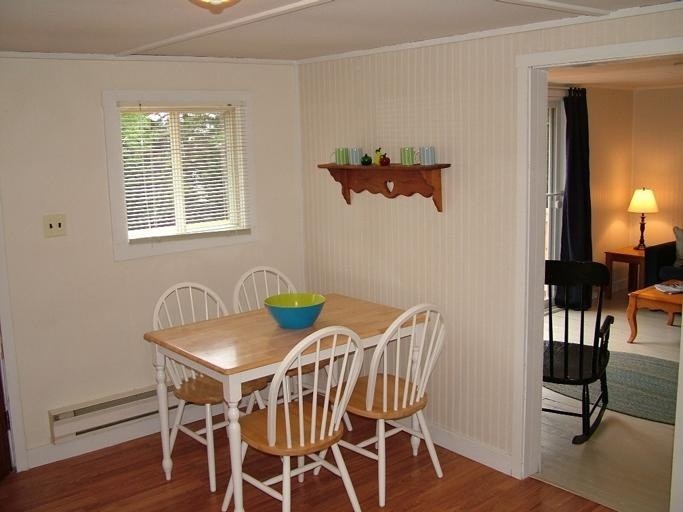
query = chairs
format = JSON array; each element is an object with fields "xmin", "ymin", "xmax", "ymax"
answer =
[
  {"xmin": 313, "ymin": 303, "xmax": 447, "ymax": 509},
  {"xmin": 643, "ymin": 240, "xmax": 682, "ymax": 316},
  {"xmin": 233, "ymin": 266, "xmax": 354, "ymax": 433},
  {"xmin": 542, "ymin": 259, "xmax": 611, "ymax": 446},
  {"xmin": 220, "ymin": 326, "xmax": 364, "ymax": 512},
  {"xmin": 153, "ymin": 281, "xmax": 284, "ymax": 492}
]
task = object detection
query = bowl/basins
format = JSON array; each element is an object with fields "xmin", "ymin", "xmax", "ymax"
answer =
[{"xmin": 262, "ymin": 291, "xmax": 327, "ymax": 330}]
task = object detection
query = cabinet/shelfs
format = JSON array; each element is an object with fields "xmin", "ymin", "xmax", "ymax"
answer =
[{"xmin": 318, "ymin": 162, "xmax": 450, "ymax": 211}]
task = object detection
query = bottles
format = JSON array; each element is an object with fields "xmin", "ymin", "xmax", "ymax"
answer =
[
  {"xmin": 379, "ymin": 153, "xmax": 390, "ymax": 166},
  {"xmin": 360, "ymin": 153, "xmax": 372, "ymax": 166},
  {"xmin": 373, "ymin": 147, "xmax": 382, "ymax": 166}
]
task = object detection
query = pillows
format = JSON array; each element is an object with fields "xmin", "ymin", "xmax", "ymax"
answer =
[{"xmin": 674, "ymin": 226, "xmax": 682, "ymax": 269}]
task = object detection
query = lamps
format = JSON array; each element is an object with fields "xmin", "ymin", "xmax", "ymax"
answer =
[
  {"xmin": 626, "ymin": 185, "xmax": 659, "ymax": 250},
  {"xmin": 188, "ymin": 0, "xmax": 242, "ymax": 17}
]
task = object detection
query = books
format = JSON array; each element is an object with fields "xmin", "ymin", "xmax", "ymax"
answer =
[{"xmin": 654, "ymin": 283, "xmax": 682, "ymax": 295}]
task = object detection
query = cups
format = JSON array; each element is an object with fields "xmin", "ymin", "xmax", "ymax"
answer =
[
  {"xmin": 329, "ymin": 147, "xmax": 349, "ymax": 165},
  {"xmin": 397, "ymin": 145, "xmax": 436, "ymax": 166},
  {"xmin": 348, "ymin": 148, "xmax": 365, "ymax": 166}
]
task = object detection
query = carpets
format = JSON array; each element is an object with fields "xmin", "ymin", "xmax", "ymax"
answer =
[{"xmin": 541, "ymin": 351, "xmax": 678, "ymax": 425}]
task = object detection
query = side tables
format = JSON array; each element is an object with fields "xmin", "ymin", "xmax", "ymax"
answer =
[{"xmin": 602, "ymin": 245, "xmax": 647, "ymax": 301}]
